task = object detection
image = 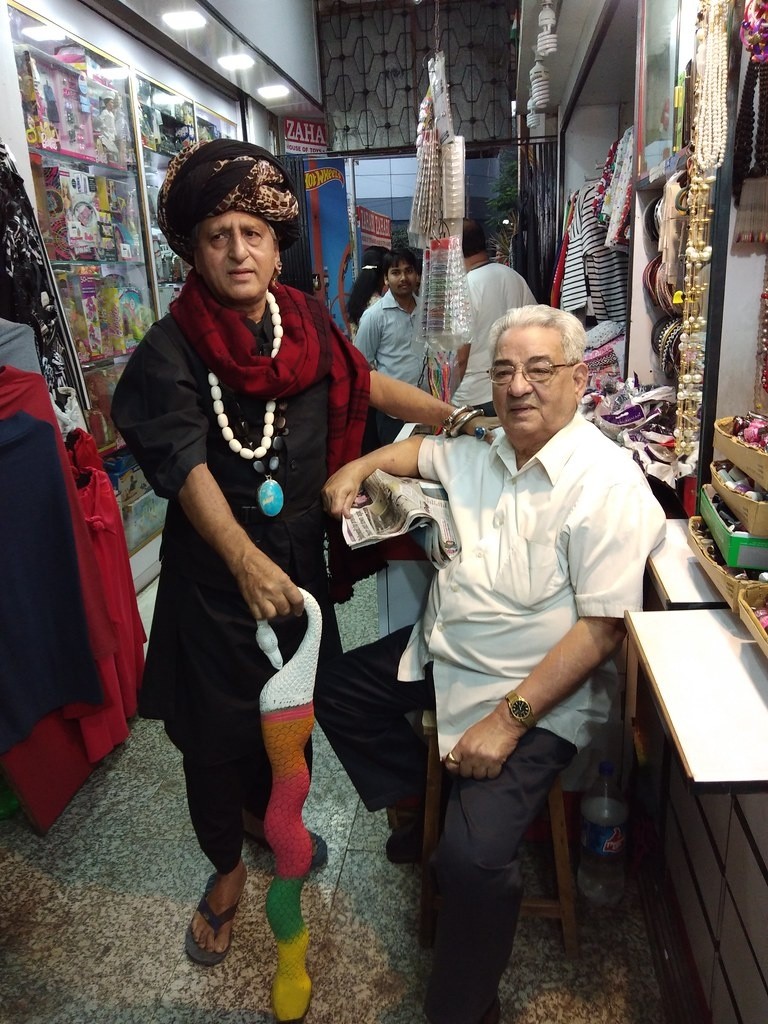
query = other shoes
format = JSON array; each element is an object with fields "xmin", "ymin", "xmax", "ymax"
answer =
[{"xmin": 386, "ymin": 789, "xmax": 452, "ymax": 863}]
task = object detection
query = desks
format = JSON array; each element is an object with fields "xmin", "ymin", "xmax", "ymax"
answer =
[{"xmin": 620, "ymin": 519, "xmax": 767, "ymax": 805}]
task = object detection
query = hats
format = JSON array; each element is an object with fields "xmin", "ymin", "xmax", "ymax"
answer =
[{"xmin": 157, "ymin": 138, "xmax": 299, "ymax": 269}]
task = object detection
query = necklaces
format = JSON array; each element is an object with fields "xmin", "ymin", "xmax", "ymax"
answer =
[
  {"xmin": 208, "ymin": 288, "xmax": 288, "ymax": 516},
  {"xmin": 674, "ymin": 0, "xmax": 768, "ymax": 456}
]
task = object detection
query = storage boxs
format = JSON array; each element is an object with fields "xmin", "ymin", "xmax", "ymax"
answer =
[
  {"xmin": 700, "ymin": 483, "xmax": 768, "ymax": 570},
  {"xmin": 710, "ymin": 458, "xmax": 768, "ymax": 536},
  {"xmin": 686, "ymin": 516, "xmax": 768, "ymax": 613},
  {"xmin": 713, "ymin": 413, "xmax": 768, "ymax": 491},
  {"xmin": 738, "ymin": 583, "xmax": 768, "ymax": 658},
  {"xmin": 23, "ymin": 56, "xmax": 175, "ymax": 554}
]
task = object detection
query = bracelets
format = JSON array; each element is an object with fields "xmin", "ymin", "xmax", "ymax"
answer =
[
  {"xmin": 449, "ymin": 409, "xmax": 484, "ymax": 438},
  {"xmin": 441, "ymin": 404, "xmax": 474, "ymax": 438}
]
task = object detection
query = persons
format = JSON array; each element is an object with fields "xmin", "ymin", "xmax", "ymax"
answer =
[
  {"xmin": 344, "ymin": 219, "xmax": 538, "ymax": 455},
  {"xmin": 111, "ymin": 140, "xmax": 502, "ymax": 965},
  {"xmin": 313, "ymin": 305, "xmax": 667, "ymax": 1024}
]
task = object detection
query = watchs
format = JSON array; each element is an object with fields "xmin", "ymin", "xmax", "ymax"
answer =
[{"xmin": 505, "ymin": 690, "xmax": 537, "ymax": 731}]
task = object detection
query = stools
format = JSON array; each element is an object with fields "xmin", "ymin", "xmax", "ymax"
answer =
[{"xmin": 420, "ymin": 706, "xmax": 586, "ymax": 956}]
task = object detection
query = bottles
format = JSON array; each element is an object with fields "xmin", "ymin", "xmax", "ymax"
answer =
[{"xmin": 576, "ymin": 762, "xmax": 632, "ymax": 909}]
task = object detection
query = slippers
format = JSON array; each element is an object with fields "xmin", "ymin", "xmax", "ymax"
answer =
[
  {"xmin": 185, "ymin": 871, "xmax": 238, "ymax": 966},
  {"xmin": 243, "ymin": 827, "xmax": 327, "ymax": 864}
]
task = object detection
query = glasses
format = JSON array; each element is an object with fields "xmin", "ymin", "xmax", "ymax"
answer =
[{"xmin": 486, "ymin": 362, "xmax": 576, "ymax": 383}]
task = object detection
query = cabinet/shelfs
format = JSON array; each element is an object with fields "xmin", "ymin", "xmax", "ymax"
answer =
[{"xmin": 0, "ymin": 0, "xmax": 238, "ymax": 598}]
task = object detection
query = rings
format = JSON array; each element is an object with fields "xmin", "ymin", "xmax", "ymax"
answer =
[
  {"xmin": 446, "ymin": 751, "xmax": 461, "ymax": 765},
  {"xmin": 475, "ymin": 427, "xmax": 490, "ymax": 440}
]
